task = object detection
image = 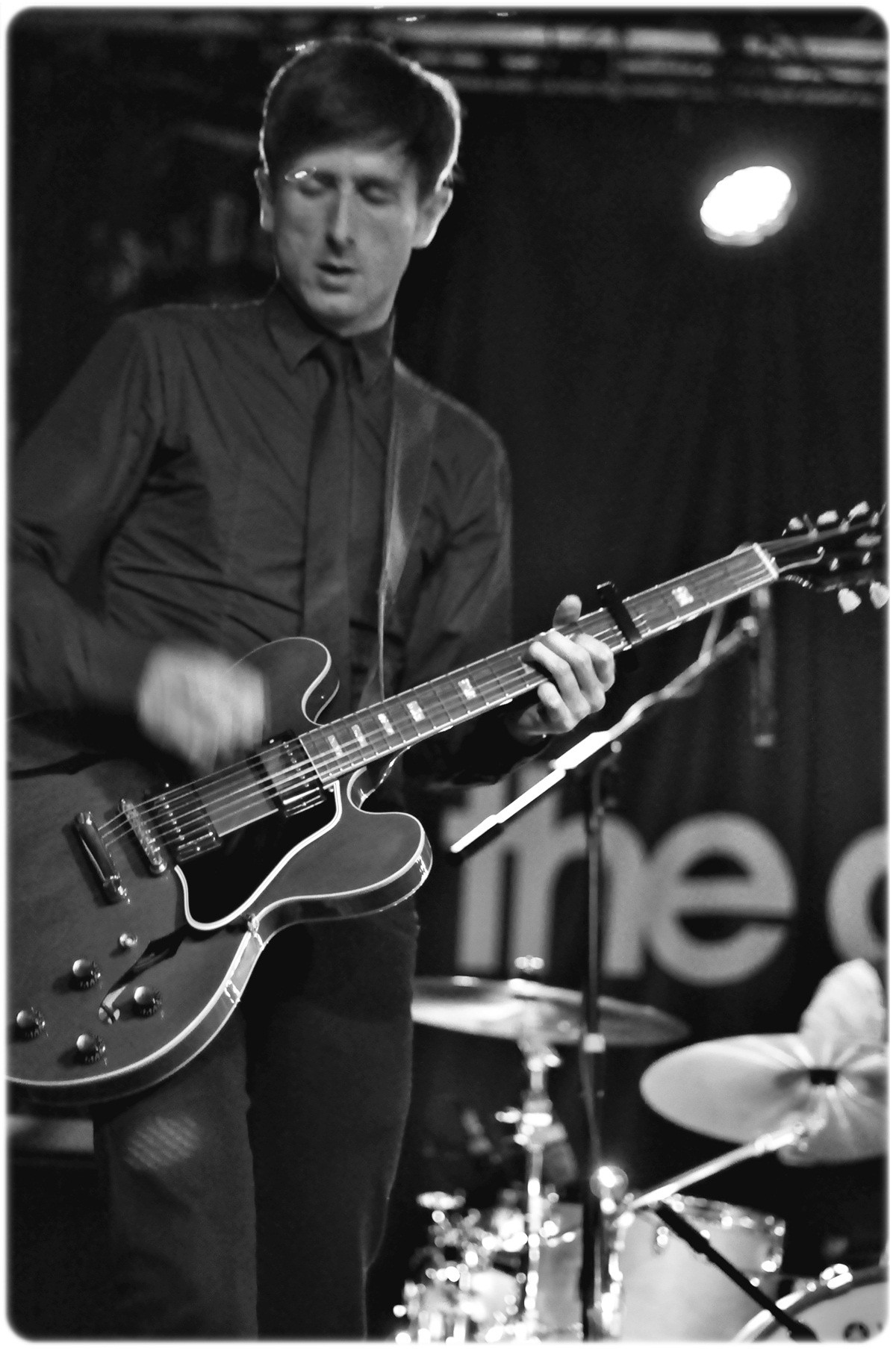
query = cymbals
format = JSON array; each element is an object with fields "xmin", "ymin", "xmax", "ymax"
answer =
[
  {"xmin": 641, "ymin": 1030, "xmax": 888, "ymax": 1165},
  {"xmin": 406, "ymin": 973, "xmax": 691, "ymax": 1048}
]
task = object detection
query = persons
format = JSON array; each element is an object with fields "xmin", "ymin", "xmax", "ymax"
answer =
[{"xmin": 5, "ymin": 33, "xmax": 616, "ymax": 1342}]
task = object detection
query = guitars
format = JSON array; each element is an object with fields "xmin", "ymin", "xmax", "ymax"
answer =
[{"xmin": 6, "ymin": 497, "xmax": 888, "ymax": 1117}]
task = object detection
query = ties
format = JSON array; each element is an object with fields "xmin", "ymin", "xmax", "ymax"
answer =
[{"xmin": 298, "ymin": 344, "xmax": 355, "ymax": 723}]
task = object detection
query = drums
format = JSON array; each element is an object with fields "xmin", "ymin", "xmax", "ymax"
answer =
[
  {"xmin": 737, "ymin": 1261, "xmax": 890, "ymax": 1340},
  {"xmin": 531, "ymin": 1188, "xmax": 783, "ymax": 1335}
]
